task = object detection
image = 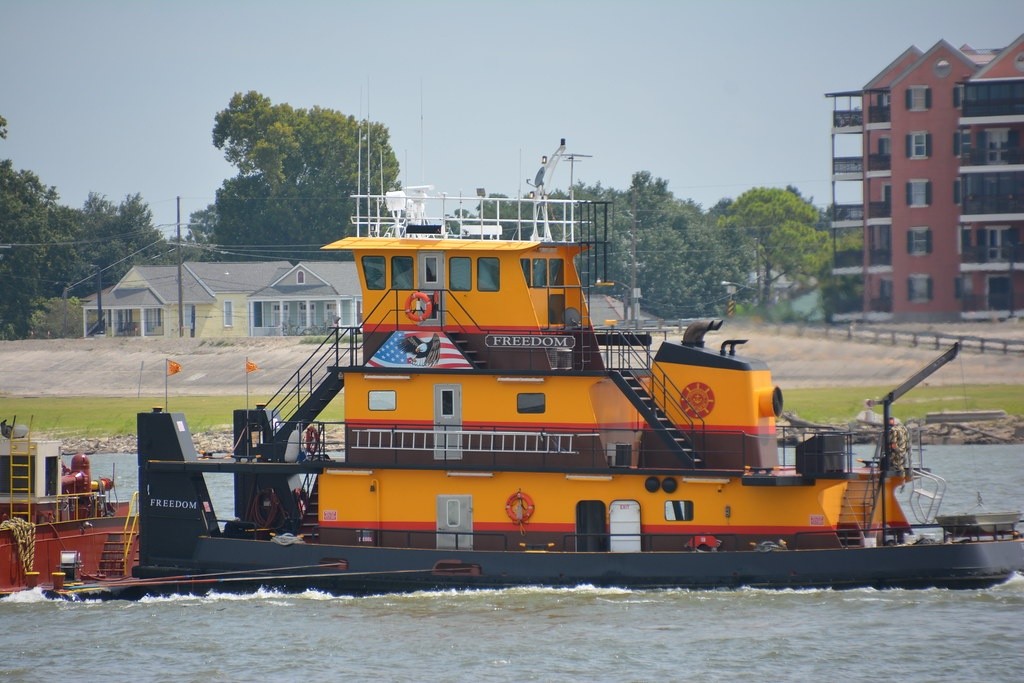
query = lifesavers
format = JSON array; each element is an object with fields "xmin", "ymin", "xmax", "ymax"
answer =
[
  {"xmin": 405, "ymin": 292, "xmax": 432, "ymax": 322},
  {"xmin": 505, "ymin": 493, "xmax": 535, "ymax": 521},
  {"xmin": 293, "ymin": 488, "xmax": 308, "ymax": 519},
  {"xmin": 306, "ymin": 426, "xmax": 319, "ymax": 453}
]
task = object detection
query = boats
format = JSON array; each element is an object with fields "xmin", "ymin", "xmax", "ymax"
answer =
[{"xmin": 131, "ymin": 80, "xmax": 1024, "ymax": 597}]
